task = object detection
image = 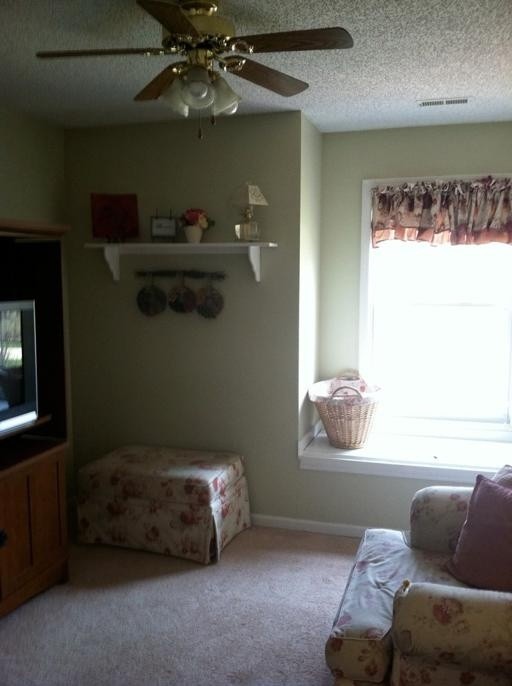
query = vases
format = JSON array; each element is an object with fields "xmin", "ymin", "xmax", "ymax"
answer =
[{"xmin": 184, "ymin": 225, "xmax": 202, "ymax": 244}]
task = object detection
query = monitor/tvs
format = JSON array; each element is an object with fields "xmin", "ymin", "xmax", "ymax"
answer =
[{"xmin": 0, "ymin": 299, "xmax": 39, "ymax": 436}]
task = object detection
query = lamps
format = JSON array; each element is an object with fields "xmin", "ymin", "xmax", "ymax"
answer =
[{"xmin": 160, "ymin": 47, "xmax": 242, "ymax": 143}]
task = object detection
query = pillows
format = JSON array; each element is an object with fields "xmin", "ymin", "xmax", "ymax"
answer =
[
  {"xmin": 447, "ymin": 474, "xmax": 512, "ymax": 594},
  {"xmin": 447, "ymin": 465, "xmax": 512, "ymax": 553}
]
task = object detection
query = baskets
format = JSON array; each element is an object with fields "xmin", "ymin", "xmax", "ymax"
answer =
[{"xmin": 314, "ymin": 367, "xmax": 377, "ymax": 449}]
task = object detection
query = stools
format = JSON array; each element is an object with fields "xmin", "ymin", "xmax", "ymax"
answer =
[{"xmin": 74, "ymin": 444, "xmax": 251, "ymax": 565}]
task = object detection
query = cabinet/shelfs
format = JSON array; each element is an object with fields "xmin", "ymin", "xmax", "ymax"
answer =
[
  {"xmin": 26, "ymin": 450, "xmax": 69, "ymax": 602},
  {"xmin": 0, "ymin": 461, "xmax": 33, "ymax": 621}
]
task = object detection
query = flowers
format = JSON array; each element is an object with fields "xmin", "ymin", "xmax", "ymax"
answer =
[{"xmin": 176, "ymin": 208, "xmax": 215, "ymax": 230}]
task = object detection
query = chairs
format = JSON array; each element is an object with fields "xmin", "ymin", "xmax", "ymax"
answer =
[{"xmin": 325, "ymin": 463, "xmax": 512, "ymax": 686}]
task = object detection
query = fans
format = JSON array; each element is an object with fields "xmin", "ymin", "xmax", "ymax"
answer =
[{"xmin": 35, "ymin": 0, "xmax": 353, "ymax": 102}]
